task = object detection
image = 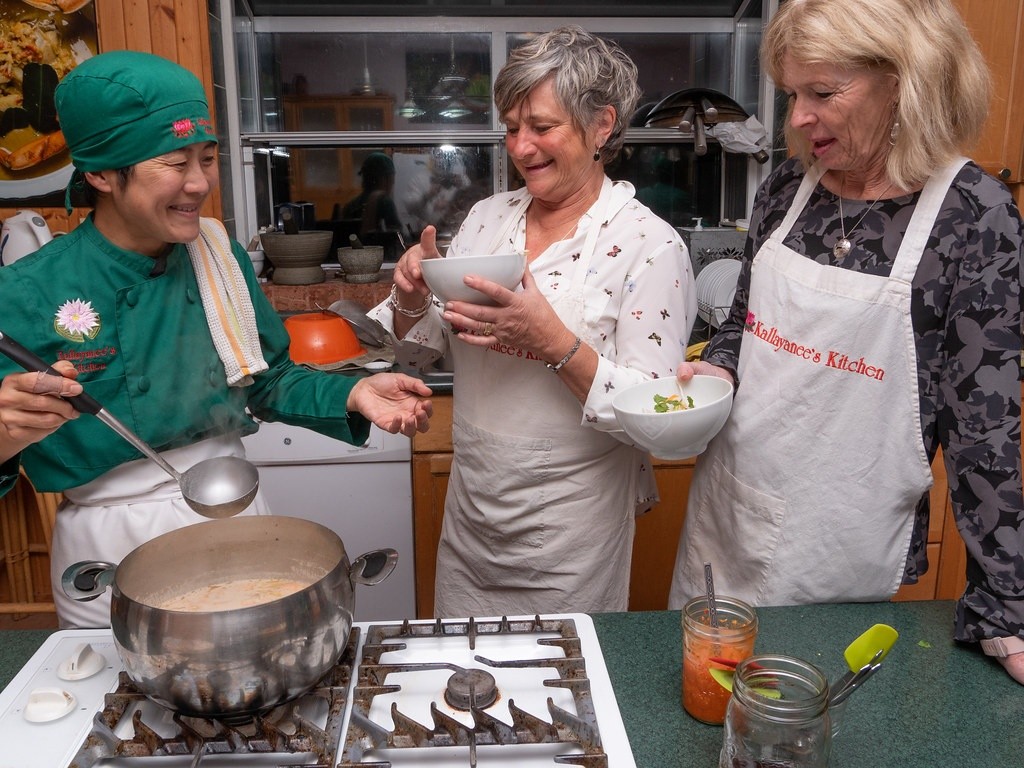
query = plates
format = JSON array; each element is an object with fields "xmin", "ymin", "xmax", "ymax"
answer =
[
  {"xmin": 364, "ymin": 361, "xmax": 393, "ymax": 374},
  {"xmin": 416, "ymin": 364, "xmax": 454, "ymax": 394},
  {"xmin": 695, "ymin": 259, "xmax": 742, "ymax": 329}
]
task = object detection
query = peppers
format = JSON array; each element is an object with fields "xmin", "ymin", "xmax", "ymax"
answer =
[{"xmin": 707, "ymin": 657, "xmax": 778, "ymax": 687}]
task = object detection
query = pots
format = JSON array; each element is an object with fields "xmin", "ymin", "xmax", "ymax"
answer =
[
  {"xmin": 60, "ymin": 515, "xmax": 398, "ymax": 719},
  {"xmin": 641, "ymin": 87, "xmax": 770, "ymax": 165}
]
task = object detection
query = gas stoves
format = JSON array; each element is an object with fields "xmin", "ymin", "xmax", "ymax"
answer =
[{"xmin": 0, "ymin": 611, "xmax": 637, "ymax": 768}]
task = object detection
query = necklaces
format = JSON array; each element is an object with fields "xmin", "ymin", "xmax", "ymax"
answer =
[{"xmin": 833, "ymin": 173, "xmax": 895, "ymax": 259}]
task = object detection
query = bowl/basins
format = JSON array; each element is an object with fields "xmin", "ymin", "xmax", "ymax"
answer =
[
  {"xmin": 283, "ymin": 312, "xmax": 368, "ymax": 366},
  {"xmin": 0, "ymin": 37, "xmax": 94, "ymax": 200},
  {"xmin": 610, "ymin": 374, "xmax": 734, "ymax": 461},
  {"xmin": 419, "ymin": 249, "xmax": 529, "ymax": 307}
]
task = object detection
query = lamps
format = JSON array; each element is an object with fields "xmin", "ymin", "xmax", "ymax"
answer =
[
  {"xmin": 436, "ymin": 35, "xmax": 471, "ymax": 92},
  {"xmin": 349, "ymin": 36, "xmax": 380, "ymax": 96},
  {"xmin": 439, "ymin": 99, "xmax": 473, "ymax": 120},
  {"xmin": 392, "ymin": 96, "xmax": 426, "ymax": 120}
]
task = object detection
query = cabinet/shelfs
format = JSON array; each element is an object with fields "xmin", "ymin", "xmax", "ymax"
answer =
[
  {"xmin": 887, "ymin": 0, "xmax": 1024, "ymax": 600},
  {"xmin": 412, "ymin": 394, "xmax": 697, "ymax": 621},
  {"xmin": 282, "ymin": 93, "xmax": 396, "ymax": 221}
]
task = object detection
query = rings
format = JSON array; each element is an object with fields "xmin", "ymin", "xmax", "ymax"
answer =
[{"xmin": 484, "ymin": 322, "xmax": 492, "ymax": 336}]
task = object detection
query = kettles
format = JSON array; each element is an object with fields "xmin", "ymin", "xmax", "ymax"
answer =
[{"xmin": 0, "ymin": 209, "xmax": 55, "ymax": 268}]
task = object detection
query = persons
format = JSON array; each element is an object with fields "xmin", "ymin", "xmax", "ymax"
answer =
[
  {"xmin": 1, "ymin": 51, "xmax": 431, "ymax": 629},
  {"xmin": 367, "ymin": 28, "xmax": 699, "ymax": 615},
  {"xmin": 678, "ymin": 2, "xmax": 1024, "ymax": 684},
  {"xmin": 345, "ymin": 153, "xmax": 402, "ymax": 240}
]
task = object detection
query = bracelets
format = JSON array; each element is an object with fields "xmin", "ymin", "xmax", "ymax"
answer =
[
  {"xmin": 390, "ymin": 283, "xmax": 433, "ymax": 317},
  {"xmin": 980, "ymin": 636, "xmax": 1024, "ymax": 656},
  {"xmin": 544, "ymin": 336, "xmax": 582, "ymax": 374}
]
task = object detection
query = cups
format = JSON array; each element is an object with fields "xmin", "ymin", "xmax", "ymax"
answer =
[{"xmin": 812, "ymin": 664, "xmax": 849, "ymax": 737}]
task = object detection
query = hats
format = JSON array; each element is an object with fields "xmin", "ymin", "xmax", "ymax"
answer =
[
  {"xmin": 358, "ymin": 152, "xmax": 394, "ymax": 177},
  {"xmin": 54, "ymin": 50, "xmax": 218, "ymax": 217}
]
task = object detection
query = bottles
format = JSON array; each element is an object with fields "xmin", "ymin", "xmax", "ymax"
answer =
[
  {"xmin": 735, "ymin": 218, "xmax": 750, "ymax": 232},
  {"xmin": 718, "ymin": 653, "xmax": 832, "ymax": 768},
  {"xmin": 681, "ymin": 594, "xmax": 760, "ymax": 726}
]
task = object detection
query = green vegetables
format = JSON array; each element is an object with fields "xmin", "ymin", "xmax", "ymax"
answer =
[{"xmin": 653, "ymin": 394, "xmax": 694, "ymax": 412}]
